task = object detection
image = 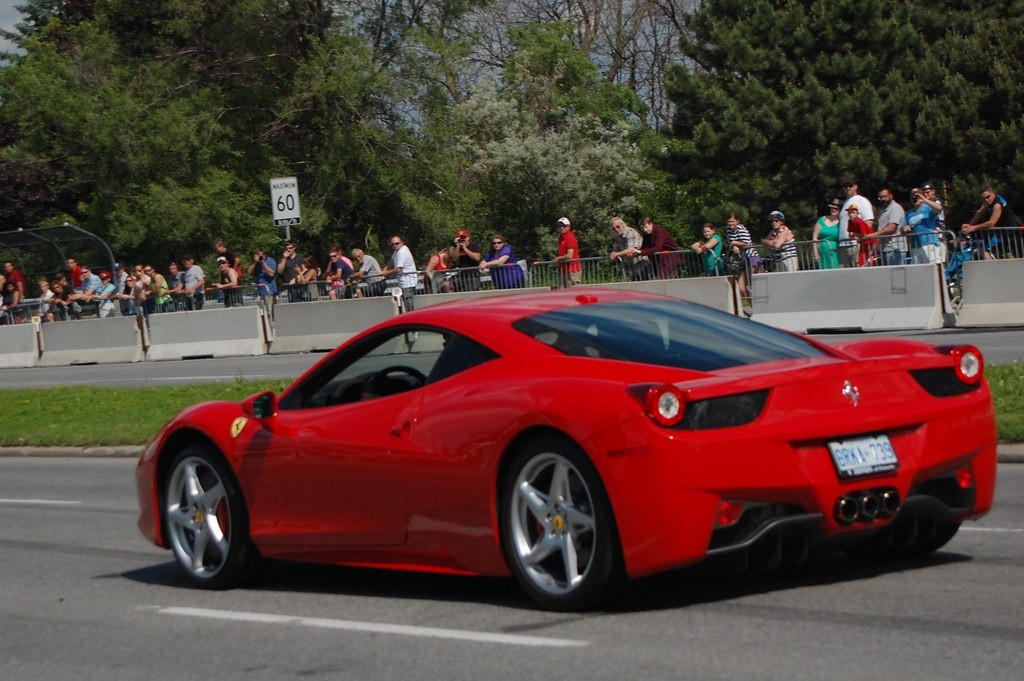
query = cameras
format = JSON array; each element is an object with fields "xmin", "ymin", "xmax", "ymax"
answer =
[
  {"xmin": 258, "ymin": 255, "xmax": 266, "ymax": 260},
  {"xmin": 454, "ymin": 235, "xmax": 467, "ymax": 242}
]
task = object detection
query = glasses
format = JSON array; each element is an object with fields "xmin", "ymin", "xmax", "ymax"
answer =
[
  {"xmin": 392, "ymin": 242, "xmax": 402, "ymax": 246},
  {"xmin": 329, "ymin": 254, "xmax": 338, "ymax": 258},
  {"xmin": 877, "ymin": 196, "xmax": 888, "ymax": 201},
  {"xmin": 491, "ymin": 241, "xmax": 502, "ymax": 245},
  {"xmin": 447, "ymin": 253, "xmax": 452, "ymax": 260},
  {"xmin": 771, "ymin": 218, "xmax": 780, "ymax": 223},
  {"xmin": 612, "ymin": 225, "xmax": 620, "ymax": 230},
  {"xmin": 844, "ymin": 185, "xmax": 854, "ymax": 188},
  {"xmin": 218, "ymin": 262, "xmax": 225, "ymax": 265},
  {"xmin": 559, "ymin": 225, "xmax": 566, "ymax": 228}
]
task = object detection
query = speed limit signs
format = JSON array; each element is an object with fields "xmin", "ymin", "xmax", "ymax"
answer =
[{"xmin": 269, "ymin": 176, "xmax": 301, "ymax": 227}]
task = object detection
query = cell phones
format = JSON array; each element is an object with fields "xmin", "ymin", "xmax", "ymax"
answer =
[{"xmin": 912, "ymin": 195, "xmax": 918, "ymax": 203}]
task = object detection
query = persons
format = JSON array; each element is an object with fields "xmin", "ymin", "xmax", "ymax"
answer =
[
  {"xmin": 609, "ymin": 216, "xmax": 682, "ymax": 282},
  {"xmin": 248, "ymin": 240, "xmax": 319, "ymax": 304},
  {"xmin": 760, "ymin": 210, "xmax": 798, "ymax": 272},
  {"xmin": 553, "ymin": 218, "xmax": 583, "ymax": 287},
  {"xmin": 812, "ymin": 173, "xmax": 1015, "ymax": 271},
  {"xmin": 213, "ymin": 238, "xmax": 243, "ymax": 307},
  {"xmin": 325, "ymin": 242, "xmax": 387, "ymax": 301},
  {"xmin": 0, "ymin": 260, "xmax": 24, "ymax": 326},
  {"xmin": 422, "ymin": 228, "xmax": 525, "ymax": 294},
  {"xmin": 377, "ymin": 236, "xmax": 418, "ymax": 310},
  {"xmin": 692, "ymin": 212, "xmax": 760, "ymax": 308},
  {"xmin": 38, "ymin": 255, "xmax": 205, "ymax": 322}
]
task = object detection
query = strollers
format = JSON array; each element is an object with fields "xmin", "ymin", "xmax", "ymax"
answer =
[{"xmin": 944, "ymin": 230, "xmax": 974, "ymax": 295}]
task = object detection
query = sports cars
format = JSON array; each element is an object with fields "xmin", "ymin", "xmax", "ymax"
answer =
[{"xmin": 132, "ymin": 289, "xmax": 996, "ymax": 614}]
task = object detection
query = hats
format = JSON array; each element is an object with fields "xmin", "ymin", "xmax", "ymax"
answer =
[
  {"xmin": 100, "ymin": 272, "xmax": 111, "ymax": 278},
  {"xmin": 217, "ymin": 256, "xmax": 228, "ymax": 263},
  {"xmin": 557, "ymin": 217, "xmax": 570, "ymax": 226},
  {"xmin": 845, "ymin": 204, "xmax": 860, "ymax": 214},
  {"xmin": 828, "ymin": 199, "xmax": 843, "ymax": 209},
  {"xmin": 114, "ymin": 263, "xmax": 124, "ymax": 270},
  {"xmin": 841, "ymin": 179, "xmax": 856, "ymax": 187},
  {"xmin": 456, "ymin": 230, "xmax": 470, "ymax": 237}
]
